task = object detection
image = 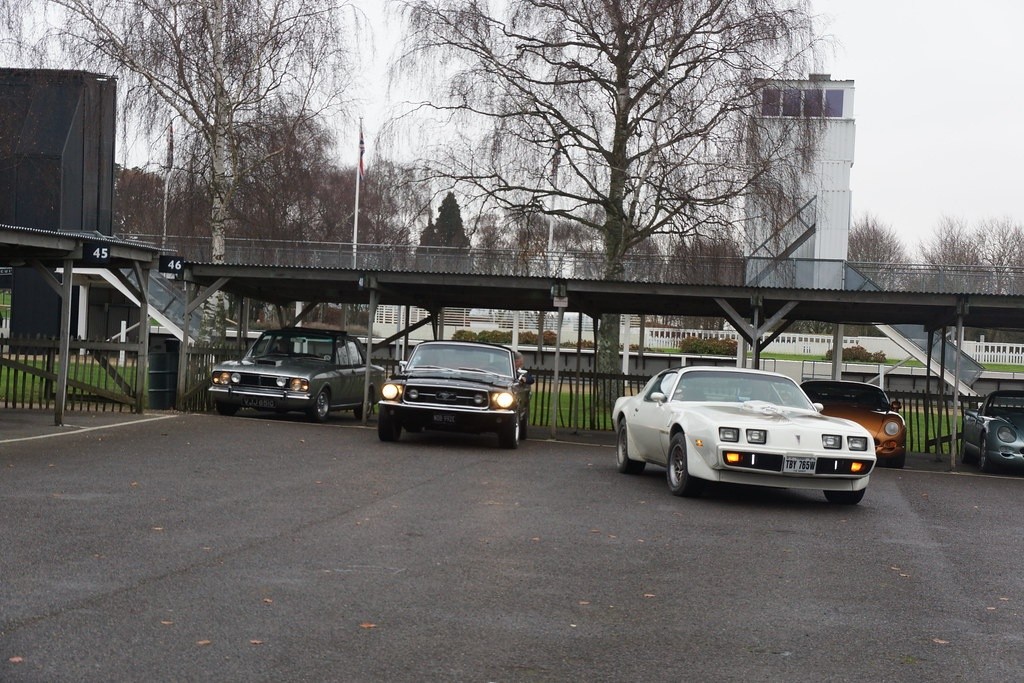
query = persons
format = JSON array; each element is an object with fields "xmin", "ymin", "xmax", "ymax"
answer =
[
  {"xmin": 487, "ymin": 351, "xmax": 534, "ymax": 384},
  {"xmin": 269, "ymin": 340, "xmax": 294, "ymax": 356}
]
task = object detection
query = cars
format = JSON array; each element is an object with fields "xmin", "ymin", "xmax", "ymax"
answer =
[
  {"xmin": 961, "ymin": 390, "xmax": 1024, "ymax": 474},
  {"xmin": 209, "ymin": 327, "xmax": 389, "ymax": 425},
  {"xmin": 375, "ymin": 340, "xmax": 536, "ymax": 449},
  {"xmin": 611, "ymin": 366, "xmax": 878, "ymax": 505},
  {"xmin": 792, "ymin": 380, "xmax": 908, "ymax": 468}
]
